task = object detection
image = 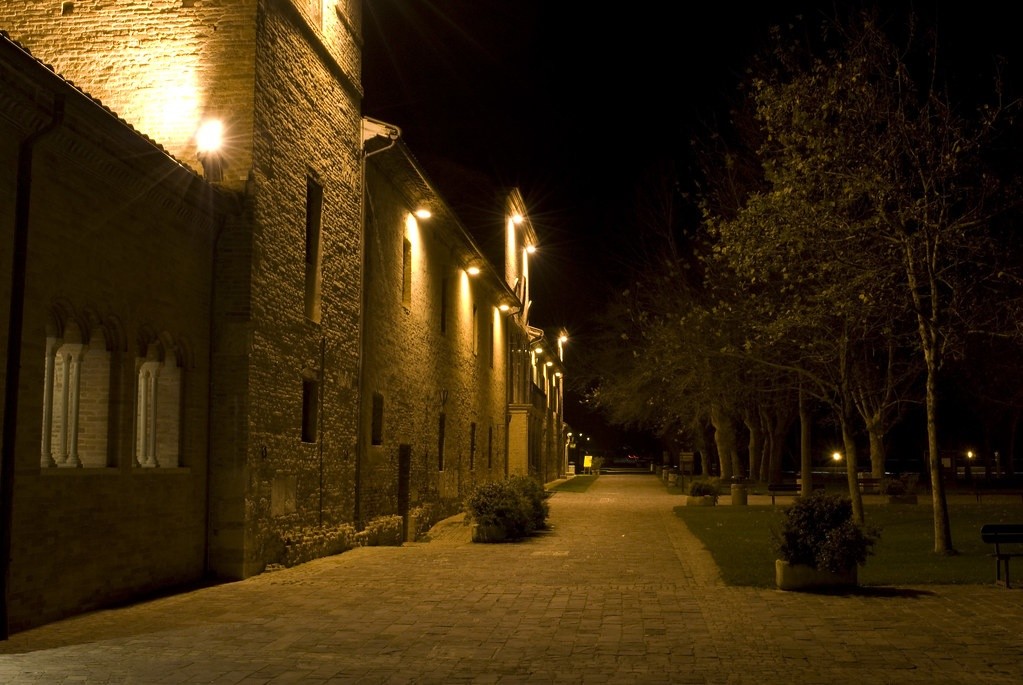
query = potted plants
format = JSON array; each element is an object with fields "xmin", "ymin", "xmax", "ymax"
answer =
[
  {"xmin": 685, "ymin": 480, "xmax": 719, "ymax": 506},
  {"xmin": 464, "ymin": 470, "xmax": 551, "ymax": 544},
  {"xmin": 884, "ymin": 479, "xmax": 919, "ymax": 504},
  {"xmin": 767, "ymin": 485, "xmax": 882, "ymax": 593}
]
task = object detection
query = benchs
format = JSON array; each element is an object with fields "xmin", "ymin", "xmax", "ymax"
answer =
[{"xmin": 980, "ymin": 523, "xmax": 1023, "ymax": 590}]
